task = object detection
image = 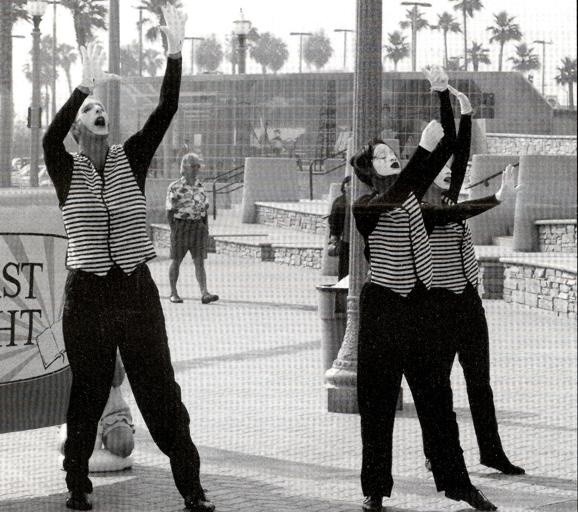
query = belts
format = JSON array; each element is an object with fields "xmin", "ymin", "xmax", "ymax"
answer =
[{"xmin": 175, "ymin": 218, "xmax": 202, "ymax": 224}]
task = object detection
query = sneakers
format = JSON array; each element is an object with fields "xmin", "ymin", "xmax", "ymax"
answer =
[
  {"xmin": 65, "ymin": 492, "xmax": 92, "ymax": 511},
  {"xmin": 185, "ymin": 488, "xmax": 216, "ymax": 512}
]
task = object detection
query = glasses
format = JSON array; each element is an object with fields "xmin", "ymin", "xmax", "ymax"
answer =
[{"xmin": 185, "ymin": 164, "xmax": 200, "ymax": 169}]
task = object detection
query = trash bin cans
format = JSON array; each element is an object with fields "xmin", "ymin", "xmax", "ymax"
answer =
[{"xmin": 315, "ymin": 286, "xmax": 349, "ymax": 369}]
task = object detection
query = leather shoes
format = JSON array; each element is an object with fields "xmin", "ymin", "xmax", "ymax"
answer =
[
  {"xmin": 171, "ymin": 296, "xmax": 183, "ymax": 303},
  {"xmin": 480, "ymin": 457, "xmax": 526, "ymax": 476},
  {"xmin": 363, "ymin": 496, "xmax": 383, "ymax": 512},
  {"xmin": 202, "ymin": 292, "xmax": 218, "ymax": 304},
  {"xmin": 445, "ymin": 489, "xmax": 498, "ymax": 512},
  {"xmin": 425, "ymin": 459, "xmax": 432, "ymax": 472}
]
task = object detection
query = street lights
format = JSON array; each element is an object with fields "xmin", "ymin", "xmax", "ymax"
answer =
[
  {"xmin": 26, "ymin": 0, "xmax": 46, "ymax": 186},
  {"xmin": 234, "ymin": 10, "xmax": 249, "ymax": 72}
]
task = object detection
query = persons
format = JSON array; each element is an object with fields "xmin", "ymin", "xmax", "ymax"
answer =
[
  {"xmin": 61, "ymin": 354, "xmax": 136, "ymax": 458},
  {"xmin": 162, "ymin": 152, "xmax": 220, "ymax": 305},
  {"xmin": 380, "ymin": 100, "xmax": 396, "ymax": 128},
  {"xmin": 40, "ymin": 4, "xmax": 218, "ymax": 512},
  {"xmin": 349, "ymin": 65, "xmax": 495, "ymax": 510},
  {"xmin": 181, "ymin": 137, "xmax": 191, "ymax": 152},
  {"xmin": 418, "ymin": 83, "xmax": 528, "ymax": 478},
  {"xmin": 325, "ymin": 170, "xmax": 351, "ymax": 282}
]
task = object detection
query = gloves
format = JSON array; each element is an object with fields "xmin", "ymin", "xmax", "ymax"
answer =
[
  {"xmin": 421, "ymin": 64, "xmax": 449, "ymax": 92},
  {"xmin": 496, "ymin": 165, "xmax": 522, "ymax": 203},
  {"xmin": 160, "ymin": 2, "xmax": 189, "ymax": 56},
  {"xmin": 80, "ymin": 42, "xmax": 123, "ymax": 96},
  {"xmin": 419, "ymin": 120, "xmax": 445, "ymax": 154},
  {"xmin": 448, "ymin": 85, "xmax": 473, "ymax": 116}
]
